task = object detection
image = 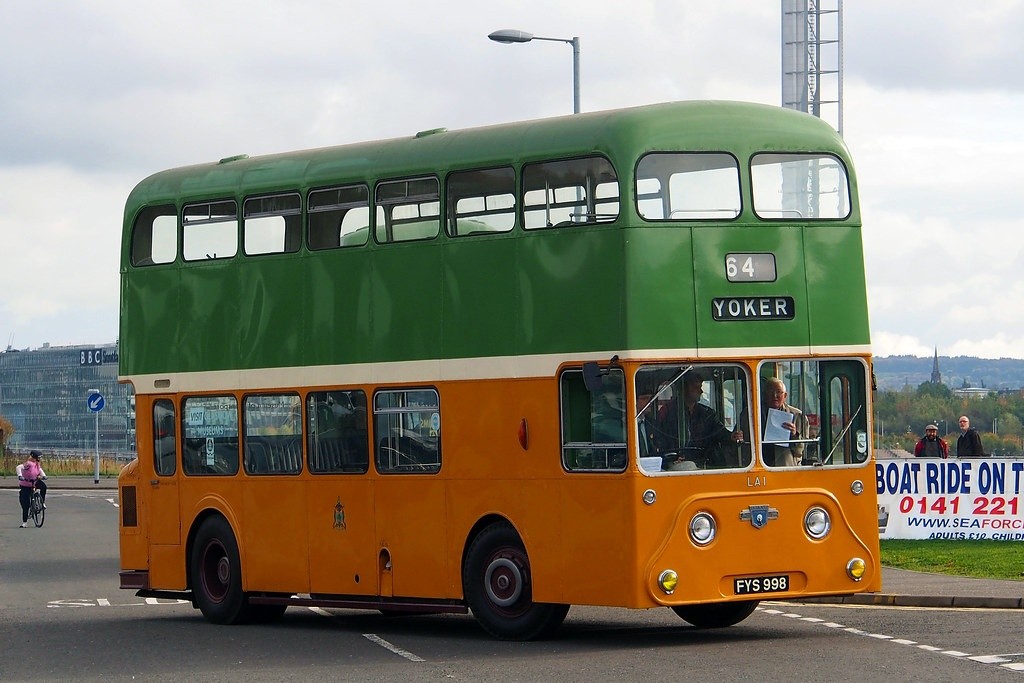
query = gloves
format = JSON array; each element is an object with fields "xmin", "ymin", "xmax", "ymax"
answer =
[
  {"xmin": 42, "ymin": 476, "xmax": 47, "ymax": 480},
  {"xmin": 19, "ymin": 476, "xmax": 24, "ymax": 481}
]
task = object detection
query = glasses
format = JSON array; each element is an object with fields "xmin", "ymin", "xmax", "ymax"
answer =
[
  {"xmin": 636, "ymin": 396, "xmax": 654, "ymax": 401},
  {"xmin": 958, "ymin": 420, "xmax": 968, "ymax": 423}
]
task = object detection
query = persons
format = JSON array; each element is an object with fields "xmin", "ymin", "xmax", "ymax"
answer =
[
  {"xmin": 914, "ymin": 425, "xmax": 948, "ymax": 459},
  {"xmin": 349, "ymin": 392, "xmax": 438, "ymax": 466},
  {"xmin": 956, "ymin": 415, "xmax": 987, "ymax": 457},
  {"xmin": 635, "ymin": 373, "xmax": 818, "ymax": 470},
  {"xmin": 16, "ymin": 449, "xmax": 48, "ymax": 528}
]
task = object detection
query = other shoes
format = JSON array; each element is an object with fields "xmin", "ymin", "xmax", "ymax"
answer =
[
  {"xmin": 20, "ymin": 522, "xmax": 27, "ymax": 528},
  {"xmin": 41, "ymin": 502, "xmax": 46, "ymax": 509}
]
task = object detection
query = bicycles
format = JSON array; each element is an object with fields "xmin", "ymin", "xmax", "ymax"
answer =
[{"xmin": 19, "ymin": 476, "xmax": 48, "ymax": 528}]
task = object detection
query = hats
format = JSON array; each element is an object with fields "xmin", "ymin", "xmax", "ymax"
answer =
[
  {"xmin": 30, "ymin": 450, "xmax": 43, "ymax": 460},
  {"xmin": 926, "ymin": 425, "xmax": 938, "ymax": 430}
]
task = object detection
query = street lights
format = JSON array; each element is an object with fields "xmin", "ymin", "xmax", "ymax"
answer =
[
  {"xmin": 941, "ymin": 420, "xmax": 947, "ymax": 435},
  {"xmin": 487, "ymin": 29, "xmax": 582, "ymax": 224}
]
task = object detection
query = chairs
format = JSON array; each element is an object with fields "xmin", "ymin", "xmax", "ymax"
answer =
[{"xmin": 192, "ymin": 429, "xmax": 425, "ymax": 472}]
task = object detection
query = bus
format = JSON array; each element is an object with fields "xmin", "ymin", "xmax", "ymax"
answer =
[{"xmin": 118, "ymin": 100, "xmax": 881, "ymax": 642}]
